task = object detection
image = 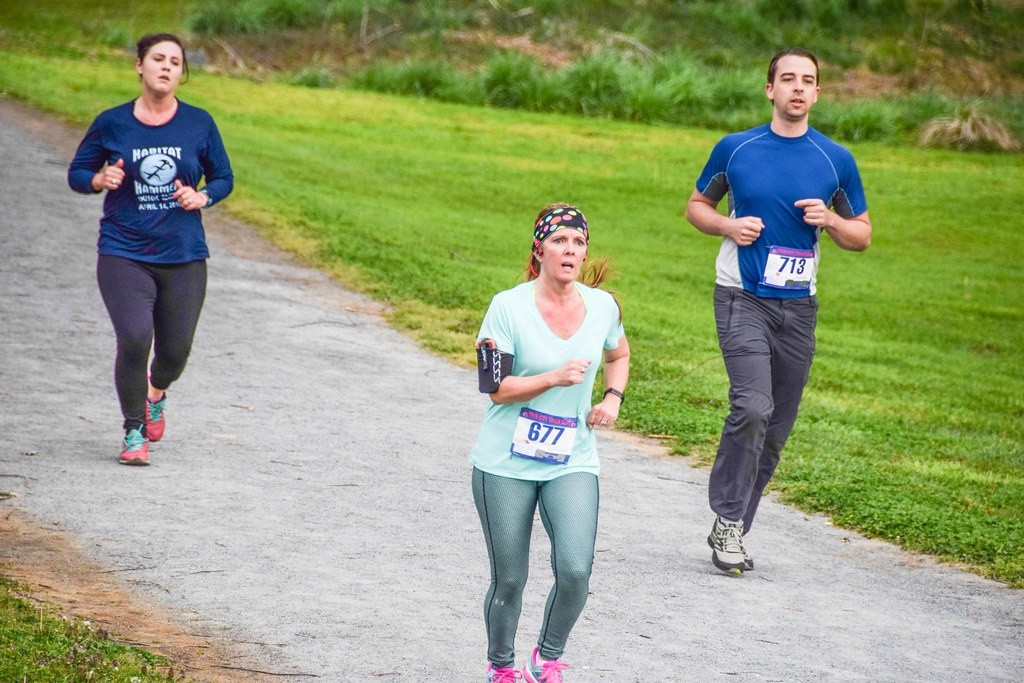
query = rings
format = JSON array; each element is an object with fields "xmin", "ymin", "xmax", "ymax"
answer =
[
  {"xmin": 600, "ymin": 420, "xmax": 608, "ymax": 427},
  {"xmin": 581, "ymin": 367, "xmax": 585, "ymax": 374},
  {"xmin": 112, "ymin": 179, "xmax": 116, "ymax": 185},
  {"xmin": 188, "ymin": 199, "xmax": 192, "ymax": 205}
]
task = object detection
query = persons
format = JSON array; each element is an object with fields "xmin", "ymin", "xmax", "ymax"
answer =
[
  {"xmin": 685, "ymin": 47, "xmax": 874, "ymax": 576},
  {"xmin": 67, "ymin": 33, "xmax": 235, "ymax": 466},
  {"xmin": 473, "ymin": 202, "xmax": 633, "ymax": 683}
]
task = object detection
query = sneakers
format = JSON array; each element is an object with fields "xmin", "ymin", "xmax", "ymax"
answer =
[
  {"xmin": 706, "ymin": 514, "xmax": 756, "ymax": 577},
  {"xmin": 486, "ymin": 646, "xmax": 564, "ymax": 683},
  {"xmin": 118, "ymin": 371, "xmax": 167, "ymax": 467}
]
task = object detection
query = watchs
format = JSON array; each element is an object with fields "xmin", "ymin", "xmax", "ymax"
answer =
[{"xmin": 604, "ymin": 388, "xmax": 626, "ymax": 406}]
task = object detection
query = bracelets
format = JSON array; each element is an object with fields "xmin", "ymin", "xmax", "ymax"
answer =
[{"xmin": 201, "ymin": 189, "xmax": 214, "ymax": 210}]
